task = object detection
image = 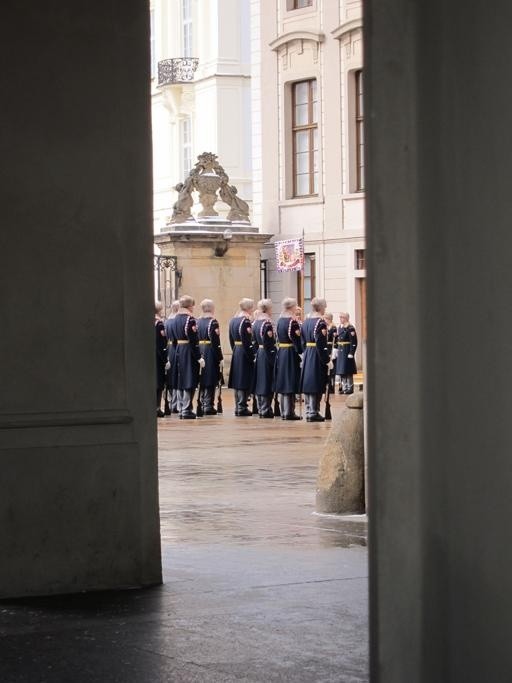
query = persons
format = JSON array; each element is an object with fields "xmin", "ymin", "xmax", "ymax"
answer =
[{"xmin": 154, "ymin": 296, "xmax": 358, "ymax": 423}]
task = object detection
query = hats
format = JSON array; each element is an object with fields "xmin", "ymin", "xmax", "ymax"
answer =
[
  {"xmin": 256, "ymin": 298, "xmax": 274, "ymax": 310},
  {"xmin": 238, "ymin": 297, "xmax": 255, "ymax": 310},
  {"xmin": 155, "ymin": 299, "xmax": 165, "ymax": 314},
  {"xmin": 323, "ymin": 311, "xmax": 333, "ymax": 320},
  {"xmin": 310, "ymin": 296, "xmax": 328, "ymax": 308},
  {"xmin": 281, "ymin": 297, "xmax": 297, "ymax": 309},
  {"xmin": 338, "ymin": 310, "xmax": 350, "ymax": 321},
  {"xmin": 179, "ymin": 294, "xmax": 196, "ymax": 308},
  {"xmin": 200, "ymin": 298, "xmax": 215, "ymax": 313},
  {"xmin": 170, "ymin": 299, "xmax": 181, "ymax": 313},
  {"xmin": 295, "ymin": 307, "xmax": 302, "ymax": 317}
]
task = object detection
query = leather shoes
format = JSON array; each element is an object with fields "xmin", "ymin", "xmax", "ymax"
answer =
[
  {"xmin": 169, "ymin": 408, "xmax": 180, "ymax": 413},
  {"xmin": 295, "ymin": 396, "xmax": 305, "ymax": 403},
  {"xmin": 201, "ymin": 406, "xmax": 218, "ymax": 415},
  {"xmin": 280, "ymin": 412, "xmax": 304, "ymax": 420},
  {"xmin": 305, "ymin": 413, "xmax": 326, "ymax": 422},
  {"xmin": 339, "ymin": 386, "xmax": 354, "ymax": 394},
  {"xmin": 258, "ymin": 411, "xmax": 275, "ymax": 418},
  {"xmin": 233, "ymin": 408, "xmax": 253, "ymax": 416},
  {"xmin": 178, "ymin": 411, "xmax": 198, "ymax": 419}
]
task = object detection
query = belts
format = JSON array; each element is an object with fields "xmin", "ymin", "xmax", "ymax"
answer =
[
  {"xmin": 198, "ymin": 340, "xmax": 212, "ymax": 344},
  {"xmin": 305, "ymin": 341, "xmax": 317, "ymax": 347},
  {"xmin": 327, "ymin": 342, "xmax": 334, "ymax": 344},
  {"xmin": 278, "ymin": 342, "xmax": 293, "ymax": 348},
  {"xmin": 176, "ymin": 338, "xmax": 190, "ymax": 344},
  {"xmin": 337, "ymin": 340, "xmax": 352, "ymax": 345},
  {"xmin": 168, "ymin": 339, "xmax": 173, "ymax": 344},
  {"xmin": 258, "ymin": 344, "xmax": 265, "ymax": 348},
  {"xmin": 234, "ymin": 340, "xmax": 243, "ymax": 345}
]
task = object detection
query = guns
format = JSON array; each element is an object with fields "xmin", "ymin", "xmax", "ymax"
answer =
[
  {"xmin": 217, "ymin": 368, "xmax": 222, "ymax": 413},
  {"xmin": 324, "ymin": 333, "xmax": 332, "ymax": 420}
]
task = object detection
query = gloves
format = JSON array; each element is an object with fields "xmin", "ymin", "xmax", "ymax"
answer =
[
  {"xmin": 197, "ymin": 356, "xmax": 208, "ymax": 368},
  {"xmin": 326, "ymin": 360, "xmax": 334, "ymax": 369},
  {"xmin": 347, "ymin": 353, "xmax": 354, "ymax": 359},
  {"xmin": 330, "ymin": 347, "xmax": 338, "ymax": 360},
  {"xmin": 219, "ymin": 359, "xmax": 225, "ymax": 372},
  {"xmin": 298, "ymin": 352, "xmax": 306, "ymax": 368},
  {"xmin": 165, "ymin": 360, "xmax": 173, "ymax": 375}
]
